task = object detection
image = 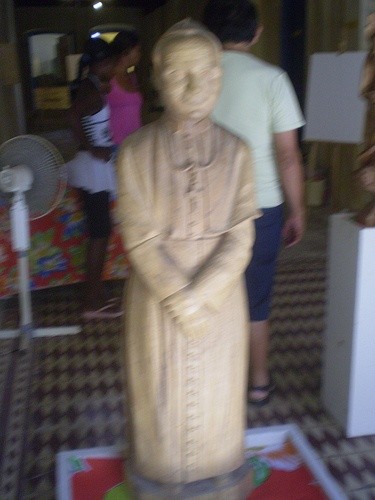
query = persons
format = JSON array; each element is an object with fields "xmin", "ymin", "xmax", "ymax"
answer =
[
  {"xmin": 69, "ymin": 38, "xmax": 127, "ymax": 319},
  {"xmin": 202, "ymin": 0, "xmax": 312, "ymax": 403},
  {"xmin": 116, "ymin": 18, "xmax": 262, "ymax": 483},
  {"xmin": 108, "ymin": 28, "xmax": 152, "ymax": 150}
]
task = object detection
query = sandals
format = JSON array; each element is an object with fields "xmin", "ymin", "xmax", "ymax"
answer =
[{"xmin": 248, "ymin": 377, "xmax": 278, "ymax": 405}]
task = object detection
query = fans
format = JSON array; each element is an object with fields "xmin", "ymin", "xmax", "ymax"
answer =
[{"xmin": 0, "ymin": 136, "xmax": 83, "ymax": 361}]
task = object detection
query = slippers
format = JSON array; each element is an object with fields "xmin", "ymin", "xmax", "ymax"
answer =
[{"xmin": 82, "ymin": 296, "xmax": 124, "ymax": 320}]
